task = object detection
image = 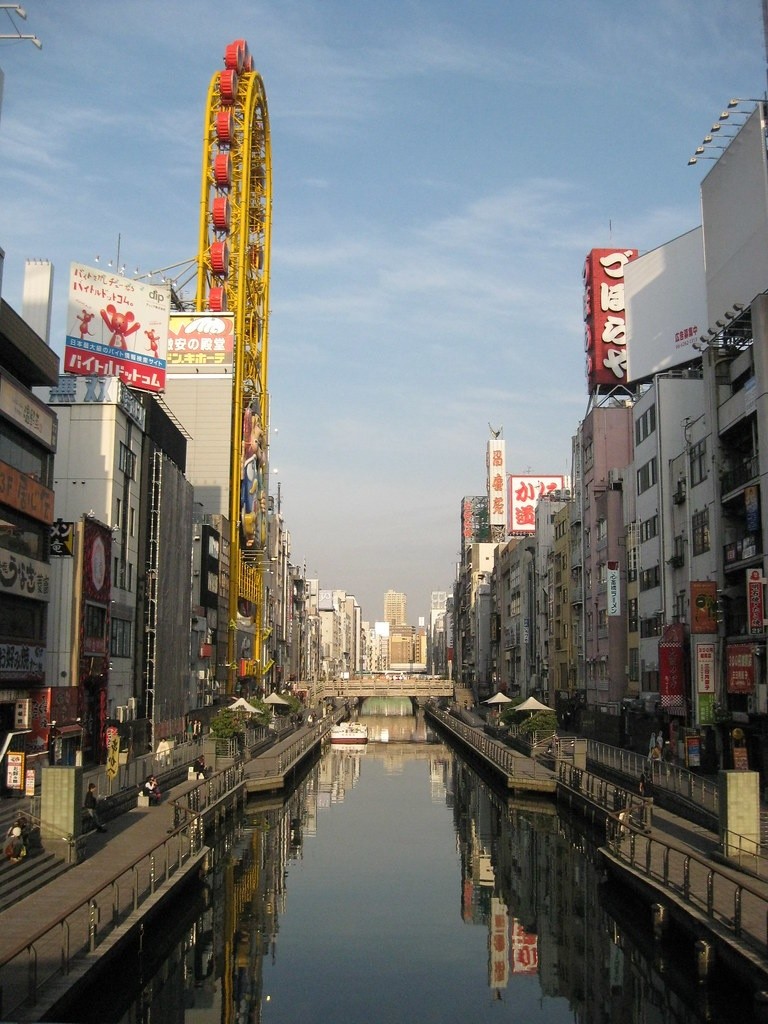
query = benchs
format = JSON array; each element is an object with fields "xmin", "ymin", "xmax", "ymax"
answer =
[
  {"xmin": 138, "ymin": 795, "xmax": 149, "ymax": 807},
  {"xmin": 188, "ymin": 771, "xmax": 199, "ymax": 780}
]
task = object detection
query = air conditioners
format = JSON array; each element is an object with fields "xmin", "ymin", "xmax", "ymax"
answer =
[
  {"xmin": 115, "ymin": 697, "xmax": 139, "ymax": 722},
  {"xmin": 748, "ymin": 684, "xmax": 767, "ymax": 715}
]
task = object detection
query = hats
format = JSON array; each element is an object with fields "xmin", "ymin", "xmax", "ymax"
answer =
[{"xmin": 13, "ymin": 827, "xmax": 21, "ymax": 836}]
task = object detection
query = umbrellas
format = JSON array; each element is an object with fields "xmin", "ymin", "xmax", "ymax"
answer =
[
  {"xmin": 260, "ymin": 693, "xmax": 289, "ymax": 717},
  {"xmin": 218, "ymin": 697, "xmax": 263, "ymax": 731},
  {"xmin": 507, "ymin": 696, "xmax": 556, "ymax": 719},
  {"xmin": 481, "ymin": 692, "xmax": 512, "ymax": 713}
]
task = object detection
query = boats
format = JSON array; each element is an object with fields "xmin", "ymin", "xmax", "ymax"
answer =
[
  {"xmin": 330, "ymin": 742, "xmax": 367, "ymax": 757},
  {"xmin": 330, "ymin": 721, "xmax": 368, "ymax": 744}
]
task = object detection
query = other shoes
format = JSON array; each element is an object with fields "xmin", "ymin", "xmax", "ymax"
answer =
[
  {"xmin": 96, "ymin": 828, "xmax": 107, "ymax": 833},
  {"xmin": 10, "ymin": 857, "xmax": 22, "ymax": 862}
]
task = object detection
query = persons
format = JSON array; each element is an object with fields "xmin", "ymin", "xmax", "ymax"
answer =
[
  {"xmin": 84, "ymin": 783, "xmax": 108, "ymax": 833},
  {"xmin": 547, "ymin": 735, "xmax": 561, "ymax": 753},
  {"xmin": 194, "ymin": 755, "xmax": 209, "ymax": 779},
  {"xmin": 155, "ymin": 738, "xmax": 171, "ymax": 767},
  {"xmin": 143, "ymin": 775, "xmax": 161, "ymax": 806},
  {"xmin": 464, "ymin": 700, "xmax": 467, "ymax": 710},
  {"xmin": 647, "ymin": 746, "xmax": 663, "ymax": 767},
  {"xmin": 5, "ymin": 817, "xmax": 32, "ymax": 863},
  {"xmin": 639, "ymin": 767, "xmax": 654, "ymax": 805},
  {"xmin": 184, "ymin": 720, "xmax": 203, "ymax": 746},
  {"xmin": 308, "ymin": 710, "xmax": 317, "ymax": 728}
]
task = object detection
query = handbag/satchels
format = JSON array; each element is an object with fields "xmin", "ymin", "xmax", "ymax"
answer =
[
  {"xmin": 5, "ymin": 841, "xmax": 22, "ymax": 859},
  {"xmin": 20, "ymin": 846, "xmax": 26, "ymax": 857}
]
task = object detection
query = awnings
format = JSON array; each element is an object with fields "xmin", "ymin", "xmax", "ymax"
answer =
[{"xmin": 56, "ymin": 724, "xmax": 83, "ymax": 739}]
